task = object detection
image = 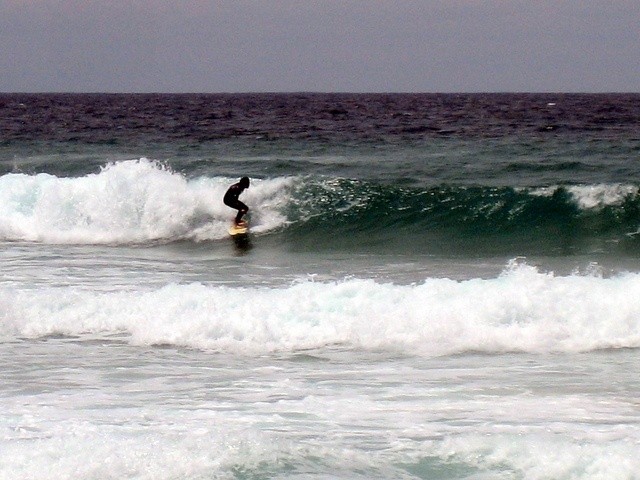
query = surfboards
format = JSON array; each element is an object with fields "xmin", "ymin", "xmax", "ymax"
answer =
[{"xmin": 227, "ymin": 221, "xmax": 248, "ymax": 236}]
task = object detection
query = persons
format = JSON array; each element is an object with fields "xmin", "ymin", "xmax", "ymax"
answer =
[{"xmin": 223, "ymin": 176, "xmax": 250, "ymax": 224}]
